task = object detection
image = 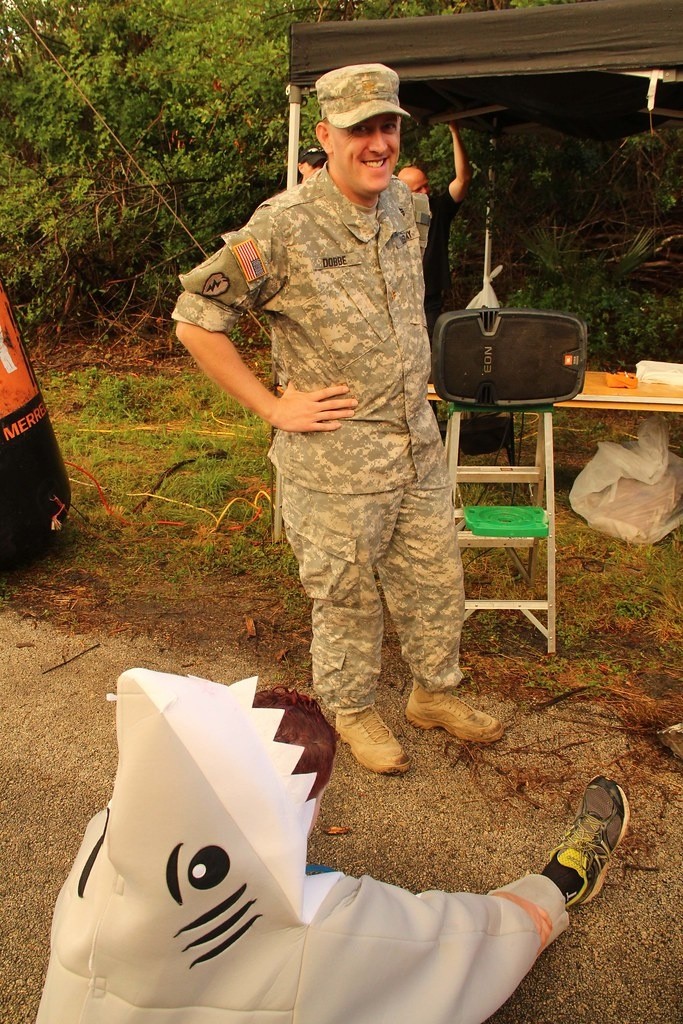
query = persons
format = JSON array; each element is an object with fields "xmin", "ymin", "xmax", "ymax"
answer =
[
  {"xmin": 33, "ymin": 666, "xmax": 633, "ymax": 1024},
  {"xmin": 398, "ymin": 120, "xmax": 471, "ymax": 331},
  {"xmin": 172, "ymin": 62, "xmax": 503, "ymax": 775},
  {"xmin": 299, "ymin": 146, "xmax": 327, "ymax": 185}
]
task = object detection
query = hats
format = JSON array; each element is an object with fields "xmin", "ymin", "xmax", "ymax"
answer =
[
  {"xmin": 315, "ymin": 63, "xmax": 411, "ymax": 128},
  {"xmin": 299, "ymin": 146, "xmax": 327, "ymax": 165}
]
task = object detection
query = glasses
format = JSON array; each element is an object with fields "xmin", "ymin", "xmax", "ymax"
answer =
[{"xmin": 301, "ymin": 147, "xmax": 323, "ymax": 158}]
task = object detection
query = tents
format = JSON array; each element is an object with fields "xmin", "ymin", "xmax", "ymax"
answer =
[{"xmin": 271, "ymin": 1, "xmax": 683, "ymax": 552}]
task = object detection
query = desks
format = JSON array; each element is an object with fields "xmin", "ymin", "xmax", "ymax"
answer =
[{"xmin": 274, "ymin": 370, "xmax": 683, "ymax": 543}]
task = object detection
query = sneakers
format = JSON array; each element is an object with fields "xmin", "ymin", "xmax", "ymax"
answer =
[
  {"xmin": 550, "ymin": 775, "xmax": 630, "ymax": 907},
  {"xmin": 404, "ymin": 678, "xmax": 503, "ymax": 743},
  {"xmin": 336, "ymin": 703, "xmax": 412, "ymax": 775}
]
